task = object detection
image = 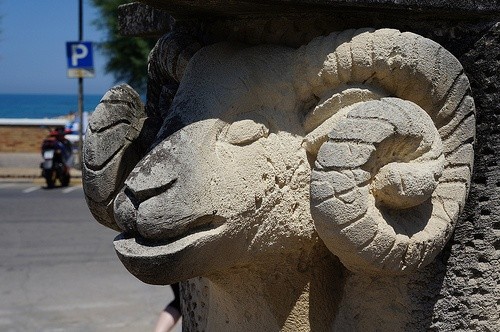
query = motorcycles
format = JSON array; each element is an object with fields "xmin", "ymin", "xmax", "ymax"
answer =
[{"xmin": 40, "ymin": 126, "xmax": 74, "ymax": 189}]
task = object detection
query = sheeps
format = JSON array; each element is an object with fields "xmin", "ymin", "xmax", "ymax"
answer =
[{"xmin": 80, "ymin": 30, "xmax": 477, "ymax": 331}]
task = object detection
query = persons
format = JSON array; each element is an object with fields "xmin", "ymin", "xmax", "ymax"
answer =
[{"xmin": 152, "ymin": 284, "xmax": 183, "ymax": 332}]
